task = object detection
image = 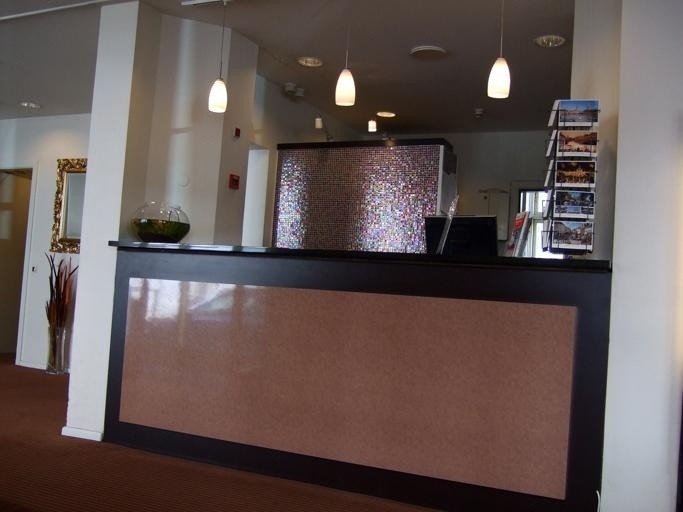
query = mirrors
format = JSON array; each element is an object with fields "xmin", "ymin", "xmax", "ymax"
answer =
[{"xmin": 48, "ymin": 155, "xmax": 87, "ymax": 255}]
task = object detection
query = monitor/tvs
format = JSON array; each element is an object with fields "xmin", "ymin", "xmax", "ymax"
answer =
[{"xmin": 425, "ymin": 216, "xmax": 497, "ymax": 257}]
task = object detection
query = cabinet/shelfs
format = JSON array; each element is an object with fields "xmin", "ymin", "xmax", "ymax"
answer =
[{"xmin": 453, "ymin": 176, "xmax": 510, "ymax": 243}]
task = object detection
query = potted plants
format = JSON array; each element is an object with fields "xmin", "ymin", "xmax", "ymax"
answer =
[{"xmin": 39, "ymin": 250, "xmax": 80, "ymax": 376}]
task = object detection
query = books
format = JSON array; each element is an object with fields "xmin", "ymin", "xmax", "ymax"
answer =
[{"xmin": 542, "ymin": 100, "xmax": 600, "ymax": 252}]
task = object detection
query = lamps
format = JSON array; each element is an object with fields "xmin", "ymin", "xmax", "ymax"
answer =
[
  {"xmin": 313, "ymin": 113, "xmax": 335, "ymax": 147},
  {"xmin": 333, "ymin": 1, "xmax": 356, "ymax": 108},
  {"xmin": 484, "ymin": 1, "xmax": 511, "ymax": 101},
  {"xmin": 206, "ymin": 0, "xmax": 229, "ymax": 117}
]
